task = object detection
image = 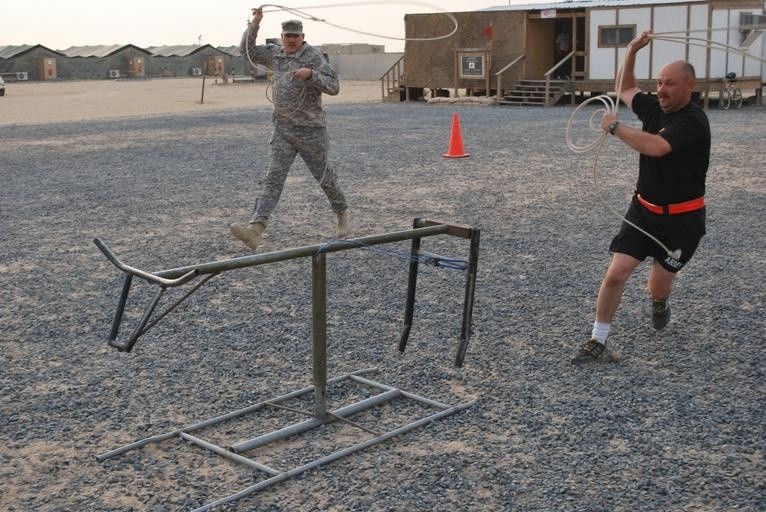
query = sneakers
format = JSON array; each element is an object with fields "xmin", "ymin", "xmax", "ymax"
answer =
[
  {"xmin": 572, "ymin": 339, "xmax": 606, "ymax": 364},
  {"xmin": 652, "ymin": 299, "xmax": 671, "ymax": 330}
]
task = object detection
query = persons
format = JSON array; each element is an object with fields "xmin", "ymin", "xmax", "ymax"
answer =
[
  {"xmin": 554, "ymin": 26, "xmax": 572, "ymax": 81},
  {"xmin": 570, "ymin": 28, "xmax": 710, "ymax": 363},
  {"xmin": 228, "ymin": 7, "xmax": 351, "ymax": 251}
]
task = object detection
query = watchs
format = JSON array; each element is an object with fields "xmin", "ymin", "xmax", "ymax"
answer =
[{"xmin": 608, "ymin": 120, "xmax": 620, "ymax": 135}]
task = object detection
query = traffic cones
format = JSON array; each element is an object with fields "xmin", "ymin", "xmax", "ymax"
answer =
[{"xmin": 442, "ymin": 113, "xmax": 469, "ymax": 157}]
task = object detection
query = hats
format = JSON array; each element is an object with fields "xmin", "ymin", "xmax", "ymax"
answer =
[{"xmin": 282, "ymin": 22, "xmax": 302, "ymax": 35}]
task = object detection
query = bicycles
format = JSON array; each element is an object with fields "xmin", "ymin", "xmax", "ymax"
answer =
[{"xmin": 718, "ymin": 77, "xmax": 742, "ymax": 110}]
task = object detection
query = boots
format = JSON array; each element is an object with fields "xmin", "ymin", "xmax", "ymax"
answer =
[
  {"xmin": 335, "ymin": 210, "xmax": 352, "ymax": 238},
  {"xmin": 230, "ymin": 223, "xmax": 264, "ymax": 249}
]
